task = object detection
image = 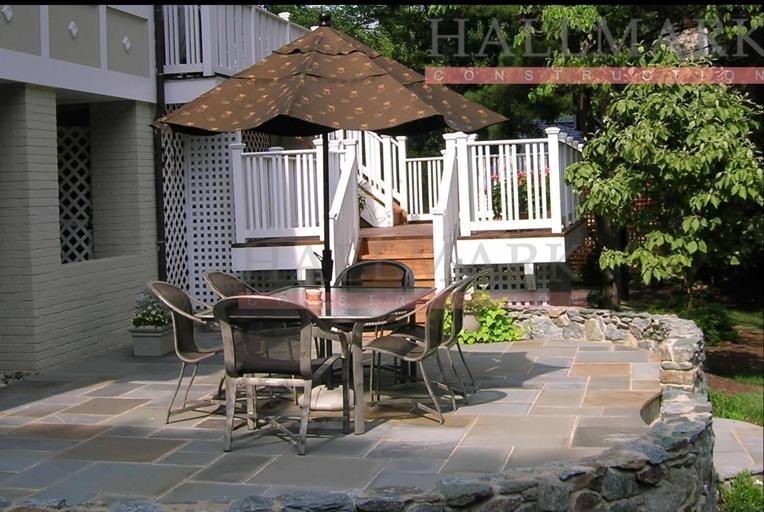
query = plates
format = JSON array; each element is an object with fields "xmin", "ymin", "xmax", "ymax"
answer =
[{"xmin": 303, "ymin": 299, "xmax": 322, "ymax": 305}]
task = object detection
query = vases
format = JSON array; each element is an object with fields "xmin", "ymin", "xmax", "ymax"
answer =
[{"xmin": 129, "ymin": 323, "xmax": 176, "ymax": 357}]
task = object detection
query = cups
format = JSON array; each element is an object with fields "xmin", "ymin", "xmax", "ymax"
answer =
[
  {"xmin": 305, "ymin": 289, "xmax": 321, "ymax": 300},
  {"xmin": 306, "ymin": 307, "xmax": 322, "ymax": 316}
]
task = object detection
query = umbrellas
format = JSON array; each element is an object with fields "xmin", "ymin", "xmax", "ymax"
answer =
[{"xmin": 149, "ymin": 11, "xmax": 509, "ymax": 392}]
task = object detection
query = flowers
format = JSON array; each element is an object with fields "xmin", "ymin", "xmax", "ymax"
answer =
[
  {"xmin": 127, "ymin": 291, "xmax": 176, "ymax": 331},
  {"xmin": 488, "ymin": 166, "xmax": 552, "ymax": 219}
]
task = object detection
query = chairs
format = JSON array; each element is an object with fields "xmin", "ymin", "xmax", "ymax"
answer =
[{"xmin": 143, "ymin": 257, "xmax": 495, "ymax": 457}]
